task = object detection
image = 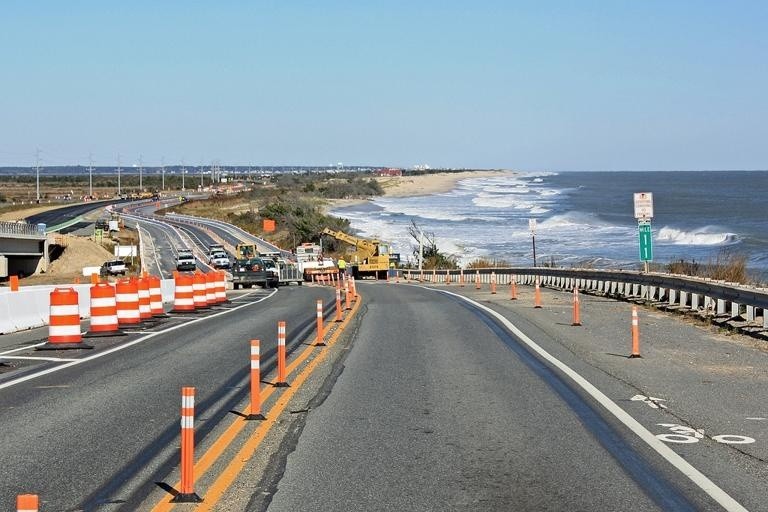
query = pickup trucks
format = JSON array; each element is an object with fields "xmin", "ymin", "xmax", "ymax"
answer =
[{"xmin": 98, "ymin": 260, "xmax": 129, "ymax": 278}]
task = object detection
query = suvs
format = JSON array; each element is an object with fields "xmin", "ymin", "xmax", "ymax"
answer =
[
  {"xmin": 258, "ymin": 250, "xmax": 293, "ymax": 288},
  {"xmin": 173, "ymin": 243, "xmax": 232, "ymax": 272},
  {"xmin": 124, "ymin": 190, "xmax": 162, "ymax": 203}
]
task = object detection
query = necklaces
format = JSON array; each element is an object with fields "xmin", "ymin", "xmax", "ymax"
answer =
[{"xmin": 336, "ymin": 255, "xmax": 346, "ymax": 278}]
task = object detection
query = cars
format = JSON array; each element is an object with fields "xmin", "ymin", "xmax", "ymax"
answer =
[{"xmin": 179, "ymin": 196, "xmax": 191, "ymax": 203}]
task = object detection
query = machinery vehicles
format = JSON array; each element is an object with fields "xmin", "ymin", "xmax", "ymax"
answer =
[
  {"xmin": 228, "ymin": 238, "xmax": 273, "ymax": 290},
  {"xmin": 295, "ymin": 243, "xmax": 342, "ymax": 282},
  {"xmin": 319, "ymin": 228, "xmax": 392, "ymax": 282}
]
